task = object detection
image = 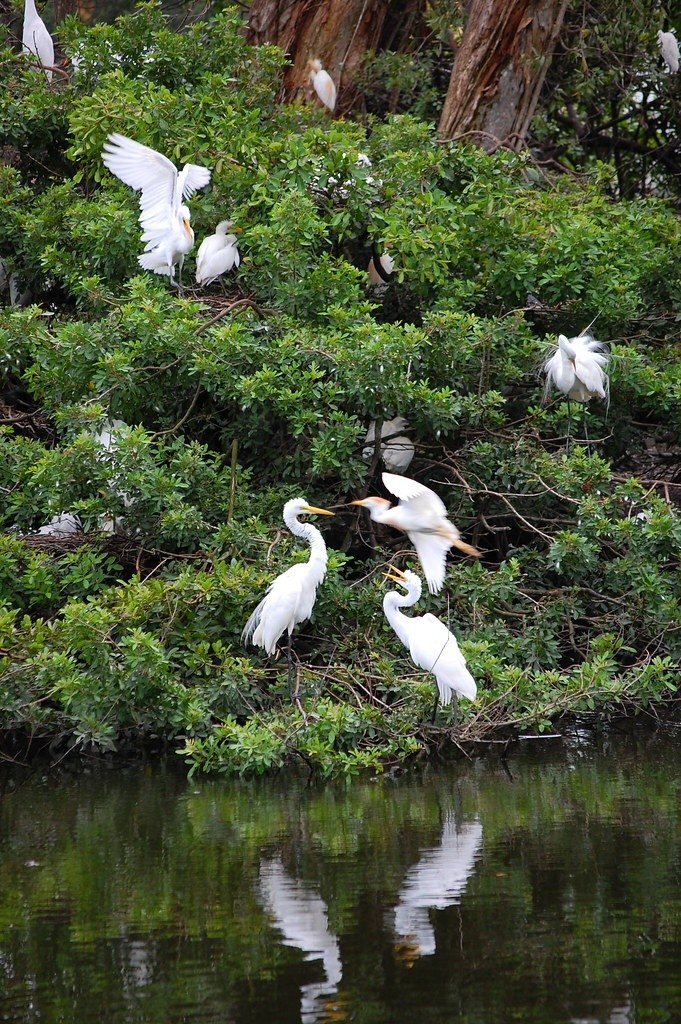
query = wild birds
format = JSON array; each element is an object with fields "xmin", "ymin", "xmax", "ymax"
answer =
[
  {"xmin": 256, "ymin": 828, "xmax": 348, "ymax": 1024},
  {"xmin": 240, "ymin": 498, "xmax": 336, "ymax": 702},
  {"xmin": 325, "ymin": 470, "xmax": 482, "ymax": 594},
  {"xmin": 392, "ymin": 787, "xmax": 486, "ymax": 968},
  {"xmin": 363, "ymin": 415, "xmax": 414, "ymax": 475},
  {"xmin": 99, "ymin": 133, "xmax": 211, "ymax": 291},
  {"xmin": 307, "ymin": 58, "xmax": 336, "ymax": 111},
  {"xmin": 656, "ymin": 30, "xmax": 681, "ymax": 71},
  {"xmin": 530, "ymin": 308, "xmax": 631, "ymax": 460},
  {"xmin": 195, "ymin": 219, "xmax": 244, "ymax": 297},
  {"xmin": 22, "ymin": 0, "xmax": 55, "ymax": 81},
  {"xmin": 380, "ymin": 563, "xmax": 478, "ymax": 725}
]
